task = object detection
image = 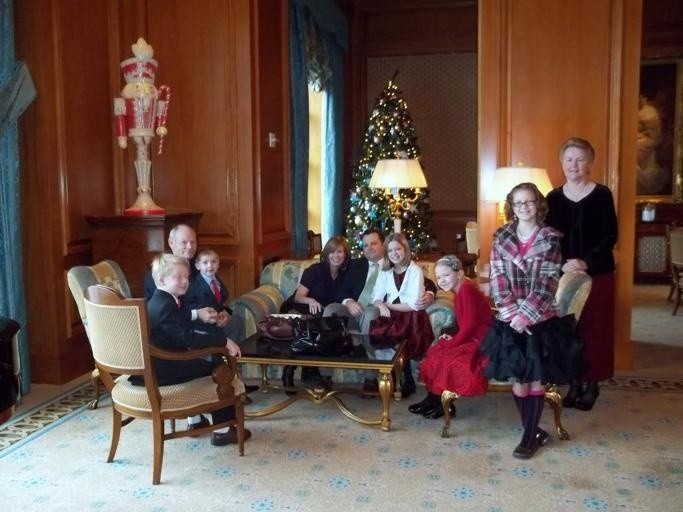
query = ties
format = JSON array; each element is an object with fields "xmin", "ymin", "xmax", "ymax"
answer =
[
  {"xmin": 176, "ymin": 299, "xmax": 182, "ymax": 309},
  {"xmin": 210, "ymin": 281, "xmax": 221, "ymax": 303},
  {"xmin": 356, "ymin": 263, "xmax": 379, "ymax": 309}
]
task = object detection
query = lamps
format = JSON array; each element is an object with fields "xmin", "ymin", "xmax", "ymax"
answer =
[
  {"xmin": 368, "ymin": 157, "xmax": 429, "ymax": 238},
  {"xmin": 484, "ymin": 160, "xmax": 554, "ymax": 227}
]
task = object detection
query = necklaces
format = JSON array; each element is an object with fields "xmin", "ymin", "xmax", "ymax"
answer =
[{"xmin": 515, "ymin": 223, "xmax": 538, "ymax": 241}]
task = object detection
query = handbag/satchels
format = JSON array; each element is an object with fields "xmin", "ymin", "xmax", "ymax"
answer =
[
  {"xmin": 257, "ymin": 314, "xmax": 304, "ymax": 341},
  {"xmin": 305, "ymin": 319, "xmax": 354, "ymax": 358},
  {"xmin": 287, "ymin": 318, "xmax": 319, "ymax": 352}
]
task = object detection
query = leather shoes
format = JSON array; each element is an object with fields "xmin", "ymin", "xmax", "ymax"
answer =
[
  {"xmin": 186, "ymin": 418, "xmax": 210, "ymax": 436},
  {"xmin": 209, "ymin": 427, "xmax": 252, "ymax": 445},
  {"xmin": 407, "ymin": 394, "xmax": 440, "ymax": 412}
]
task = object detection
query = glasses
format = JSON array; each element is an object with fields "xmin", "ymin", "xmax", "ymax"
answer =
[{"xmin": 512, "ymin": 201, "xmax": 535, "ymax": 207}]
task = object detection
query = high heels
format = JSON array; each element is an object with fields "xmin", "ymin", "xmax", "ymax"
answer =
[{"xmin": 423, "ymin": 402, "xmax": 457, "ymax": 420}]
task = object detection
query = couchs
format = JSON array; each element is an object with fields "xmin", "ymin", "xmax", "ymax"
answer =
[{"xmin": 229, "ymin": 258, "xmax": 457, "ymax": 389}]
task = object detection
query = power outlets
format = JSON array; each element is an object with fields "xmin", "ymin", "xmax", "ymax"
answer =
[{"xmin": 268, "ymin": 133, "xmax": 276, "ymax": 146}]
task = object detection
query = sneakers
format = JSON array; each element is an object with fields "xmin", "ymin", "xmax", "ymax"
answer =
[
  {"xmin": 512, "ymin": 442, "xmax": 540, "ymax": 459},
  {"xmin": 281, "ymin": 375, "xmax": 298, "ymax": 396},
  {"xmin": 535, "ymin": 429, "xmax": 551, "ymax": 446},
  {"xmin": 362, "ymin": 380, "xmax": 378, "ymax": 399},
  {"xmin": 577, "ymin": 384, "xmax": 598, "ymax": 409},
  {"xmin": 563, "ymin": 383, "xmax": 582, "ymax": 406},
  {"xmin": 242, "ymin": 384, "xmax": 261, "ymax": 393}
]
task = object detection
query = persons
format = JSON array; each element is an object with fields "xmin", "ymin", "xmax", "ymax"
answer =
[
  {"xmin": 489, "ymin": 182, "xmax": 565, "ymax": 460},
  {"xmin": 408, "ymin": 255, "xmax": 494, "ymax": 420},
  {"xmin": 279, "ymin": 235, "xmax": 352, "ymax": 397},
  {"xmin": 184, "ymin": 246, "xmax": 233, "ymax": 333},
  {"xmin": 371, "ymin": 234, "xmax": 435, "ymax": 398},
  {"xmin": 127, "ymin": 254, "xmax": 252, "ymax": 446},
  {"xmin": 314, "ymin": 229, "xmax": 437, "ymax": 399},
  {"xmin": 545, "ymin": 137, "xmax": 618, "ymax": 412},
  {"xmin": 142, "ymin": 224, "xmax": 260, "ymax": 407},
  {"xmin": 637, "ymin": 93, "xmax": 669, "ymax": 196}
]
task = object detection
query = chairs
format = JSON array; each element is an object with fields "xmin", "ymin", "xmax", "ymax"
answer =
[
  {"xmin": 65, "ymin": 259, "xmax": 138, "ymax": 404},
  {"xmin": 81, "ymin": 284, "xmax": 245, "ymax": 488},
  {"xmin": 444, "ymin": 265, "xmax": 593, "ymax": 429},
  {"xmin": 661, "ymin": 224, "xmax": 683, "ymax": 316}
]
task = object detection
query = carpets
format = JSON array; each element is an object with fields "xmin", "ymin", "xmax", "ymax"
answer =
[{"xmin": 0, "ymin": 371, "xmax": 679, "ymax": 512}]
